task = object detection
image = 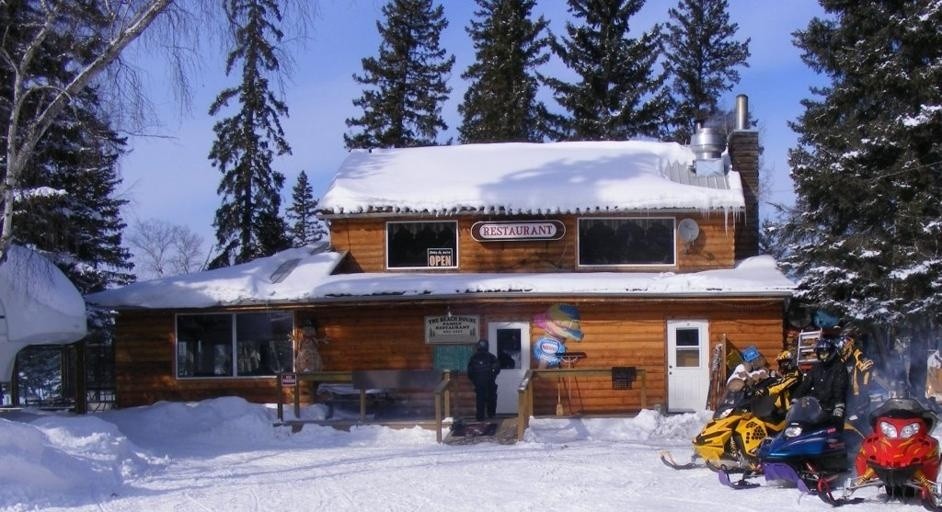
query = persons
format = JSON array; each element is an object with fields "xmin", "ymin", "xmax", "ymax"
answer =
[
  {"xmin": 756, "ymin": 348, "xmax": 805, "ymax": 415},
  {"xmin": 467, "ymin": 339, "xmax": 501, "ymax": 419},
  {"xmin": 793, "ymin": 338, "xmax": 850, "ymax": 431},
  {"xmin": 726, "ymin": 359, "xmax": 752, "ymax": 391},
  {"xmin": 745, "ymin": 354, "xmax": 770, "ymax": 389}
]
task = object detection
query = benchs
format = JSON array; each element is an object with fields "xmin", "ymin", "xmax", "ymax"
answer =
[{"xmin": 313, "ymin": 383, "xmax": 394, "ymax": 422}]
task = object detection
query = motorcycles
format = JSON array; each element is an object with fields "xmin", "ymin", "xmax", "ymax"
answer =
[
  {"xmin": 819, "ymin": 398, "xmax": 942, "ymax": 511},
  {"xmin": 658, "ymin": 371, "xmax": 803, "ymax": 477},
  {"xmin": 718, "ymin": 392, "xmax": 876, "ymax": 493}
]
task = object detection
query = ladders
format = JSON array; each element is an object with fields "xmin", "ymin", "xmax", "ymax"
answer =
[{"xmin": 797, "ymin": 328, "xmax": 823, "ymax": 366}]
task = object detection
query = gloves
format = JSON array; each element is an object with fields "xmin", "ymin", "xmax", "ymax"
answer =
[{"xmin": 833, "ymin": 403, "xmax": 846, "ymax": 419}]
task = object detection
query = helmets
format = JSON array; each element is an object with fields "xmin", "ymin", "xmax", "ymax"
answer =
[
  {"xmin": 475, "ymin": 340, "xmax": 487, "ymax": 350},
  {"xmin": 775, "ymin": 350, "xmax": 792, "ymax": 362},
  {"xmin": 813, "ymin": 338, "xmax": 837, "ymax": 363}
]
